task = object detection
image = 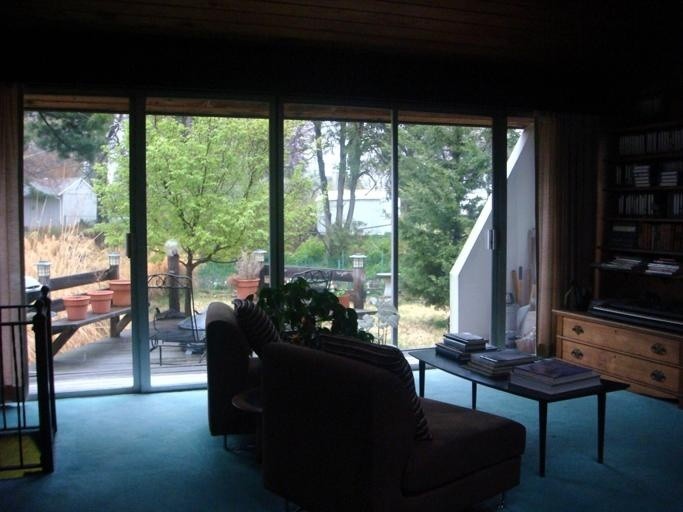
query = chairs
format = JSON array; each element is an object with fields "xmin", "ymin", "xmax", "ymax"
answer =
[
  {"xmin": 290, "ymin": 269, "xmax": 333, "ymax": 289},
  {"xmin": 147, "ymin": 272, "xmax": 208, "ymax": 363},
  {"xmin": 206, "ymin": 301, "xmax": 267, "ymax": 449},
  {"xmin": 258, "ymin": 341, "xmax": 524, "ymax": 511}
]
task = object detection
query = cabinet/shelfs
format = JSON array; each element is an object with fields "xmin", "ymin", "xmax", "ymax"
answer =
[{"xmin": 551, "ymin": 121, "xmax": 683, "ymax": 407}]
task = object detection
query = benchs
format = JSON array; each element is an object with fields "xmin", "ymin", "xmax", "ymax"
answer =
[{"xmin": 46, "ymin": 268, "xmax": 150, "ymax": 356}]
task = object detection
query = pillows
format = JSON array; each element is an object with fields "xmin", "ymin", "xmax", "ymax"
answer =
[
  {"xmin": 234, "ymin": 296, "xmax": 282, "ymax": 360},
  {"xmin": 318, "ymin": 333, "xmax": 432, "ymax": 443}
]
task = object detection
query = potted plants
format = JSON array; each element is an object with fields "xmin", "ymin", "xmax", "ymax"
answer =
[{"xmin": 332, "ymin": 287, "xmax": 351, "ymax": 308}]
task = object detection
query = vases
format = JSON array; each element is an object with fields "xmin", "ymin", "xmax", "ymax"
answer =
[
  {"xmin": 63, "ymin": 296, "xmax": 92, "ymax": 320},
  {"xmin": 88, "ymin": 290, "xmax": 115, "ymax": 314},
  {"xmin": 234, "ymin": 277, "xmax": 261, "ymax": 300},
  {"xmin": 110, "ymin": 280, "xmax": 132, "ymax": 307}
]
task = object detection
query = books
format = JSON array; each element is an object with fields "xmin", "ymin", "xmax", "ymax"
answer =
[
  {"xmin": 435, "ymin": 331, "xmax": 602, "ymax": 395},
  {"xmin": 606, "ymin": 131, "xmax": 683, "ymax": 276}
]
